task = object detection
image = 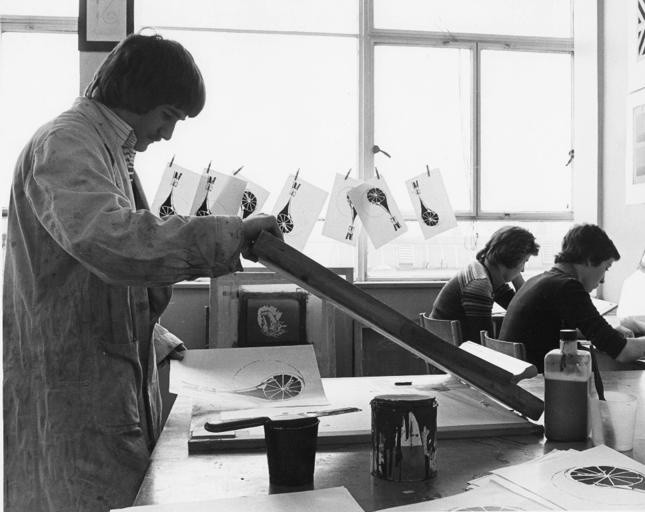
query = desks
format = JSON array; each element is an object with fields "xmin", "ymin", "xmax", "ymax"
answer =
[{"xmin": 122, "ymin": 369, "xmax": 645, "ymax": 512}]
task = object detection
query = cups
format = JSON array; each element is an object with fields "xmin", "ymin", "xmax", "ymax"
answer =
[
  {"xmin": 264, "ymin": 418, "xmax": 319, "ymax": 484},
  {"xmin": 589, "ymin": 391, "xmax": 637, "ymax": 452}
]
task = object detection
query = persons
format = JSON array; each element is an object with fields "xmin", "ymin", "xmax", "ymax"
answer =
[
  {"xmin": 432, "ymin": 225, "xmax": 539, "ymax": 372},
  {"xmin": 4, "ymin": 33, "xmax": 283, "ymax": 512},
  {"xmin": 618, "ymin": 247, "xmax": 645, "ymax": 335},
  {"xmin": 501, "ymin": 220, "xmax": 645, "ymax": 372}
]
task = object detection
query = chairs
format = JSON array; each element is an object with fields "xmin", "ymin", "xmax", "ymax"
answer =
[
  {"xmin": 479, "ymin": 329, "xmax": 526, "ymax": 361},
  {"xmin": 418, "ymin": 312, "xmax": 464, "ymax": 373}
]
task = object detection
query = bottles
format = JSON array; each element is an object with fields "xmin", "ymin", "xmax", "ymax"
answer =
[{"xmin": 544, "ymin": 329, "xmax": 593, "ymax": 442}]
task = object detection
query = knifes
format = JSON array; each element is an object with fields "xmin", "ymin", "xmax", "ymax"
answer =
[{"xmin": 204, "ymin": 407, "xmax": 363, "ymax": 432}]
